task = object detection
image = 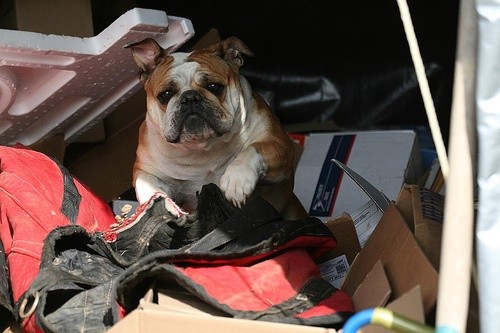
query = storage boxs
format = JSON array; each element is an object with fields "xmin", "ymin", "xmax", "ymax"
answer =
[
  {"xmin": 293, "ymin": 128, "xmax": 423, "ymax": 253},
  {"xmin": 94, "ymin": 292, "xmax": 337, "ymax": 333},
  {"xmin": 61, "ymin": 81, "xmax": 153, "ymax": 210},
  {"xmin": 395, "ymin": 183, "xmax": 445, "ymax": 269},
  {"xmin": 5, "ymin": 1, "xmax": 107, "ymax": 145}
]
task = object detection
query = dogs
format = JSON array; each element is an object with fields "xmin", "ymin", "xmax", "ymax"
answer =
[{"xmin": 123, "ymin": 37, "xmax": 299, "ymax": 217}]
